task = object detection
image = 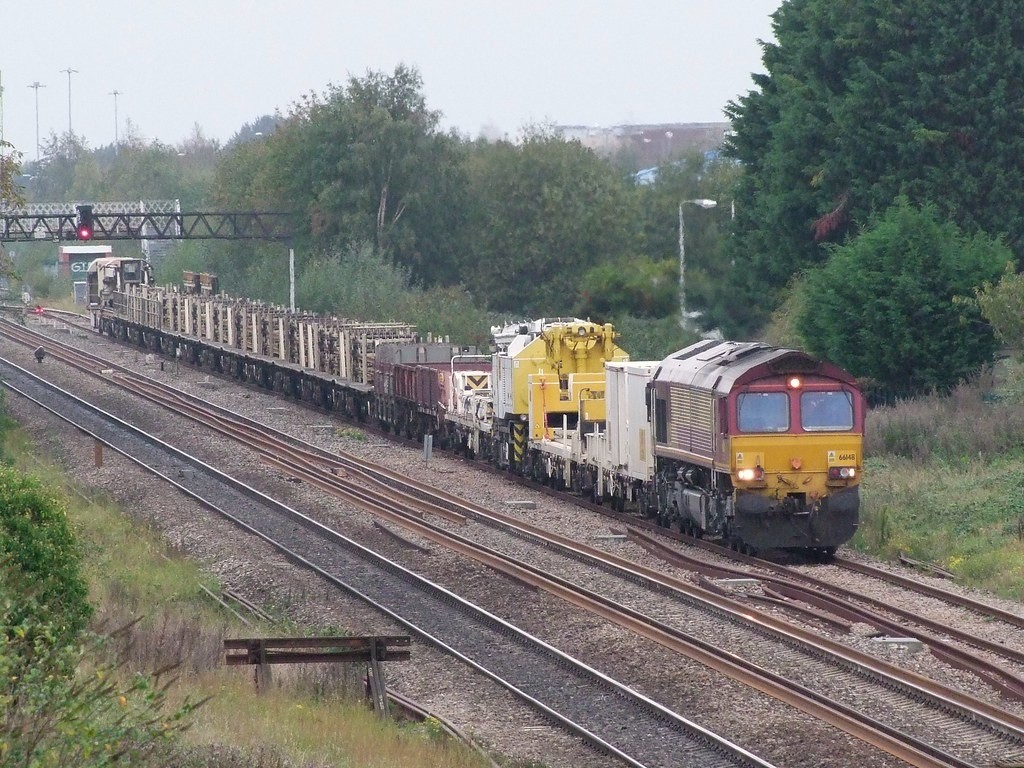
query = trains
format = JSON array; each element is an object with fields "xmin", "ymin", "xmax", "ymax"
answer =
[{"xmin": 87, "ymin": 256, "xmax": 866, "ymax": 560}]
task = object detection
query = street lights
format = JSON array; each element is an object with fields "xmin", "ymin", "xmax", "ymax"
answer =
[{"xmin": 678, "ymin": 197, "xmax": 719, "ymax": 320}]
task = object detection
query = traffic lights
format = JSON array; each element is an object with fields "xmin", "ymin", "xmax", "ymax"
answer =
[{"xmin": 76, "ymin": 206, "xmax": 93, "ymax": 241}]
